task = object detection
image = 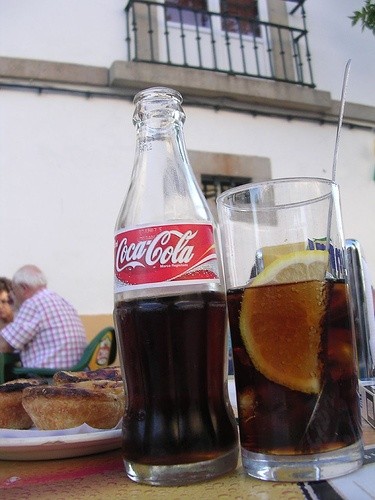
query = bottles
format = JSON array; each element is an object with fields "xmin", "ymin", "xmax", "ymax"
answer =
[{"xmin": 111, "ymin": 86, "xmax": 241, "ymax": 488}]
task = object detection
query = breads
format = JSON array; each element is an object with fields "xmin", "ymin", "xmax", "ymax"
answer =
[{"xmin": 0, "ymin": 365, "xmax": 126, "ymax": 432}]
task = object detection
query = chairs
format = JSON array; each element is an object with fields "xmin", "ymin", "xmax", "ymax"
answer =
[{"xmin": 13, "ymin": 327, "xmax": 117, "ymax": 379}]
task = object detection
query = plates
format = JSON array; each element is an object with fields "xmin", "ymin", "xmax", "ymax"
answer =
[{"xmin": 0, "ymin": 374, "xmax": 239, "ymax": 460}]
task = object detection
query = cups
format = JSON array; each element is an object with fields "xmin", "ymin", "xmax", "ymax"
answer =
[{"xmin": 215, "ymin": 176, "xmax": 366, "ymax": 484}]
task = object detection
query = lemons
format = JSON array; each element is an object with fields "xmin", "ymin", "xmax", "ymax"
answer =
[{"xmin": 239, "ymin": 249, "xmax": 329, "ymax": 393}]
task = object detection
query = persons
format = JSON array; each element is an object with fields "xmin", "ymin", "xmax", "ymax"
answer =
[
  {"xmin": 0, "ymin": 276, "xmax": 17, "ymax": 328},
  {"xmin": 0, "ymin": 263, "xmax": 88, "ymax": 376}
]
